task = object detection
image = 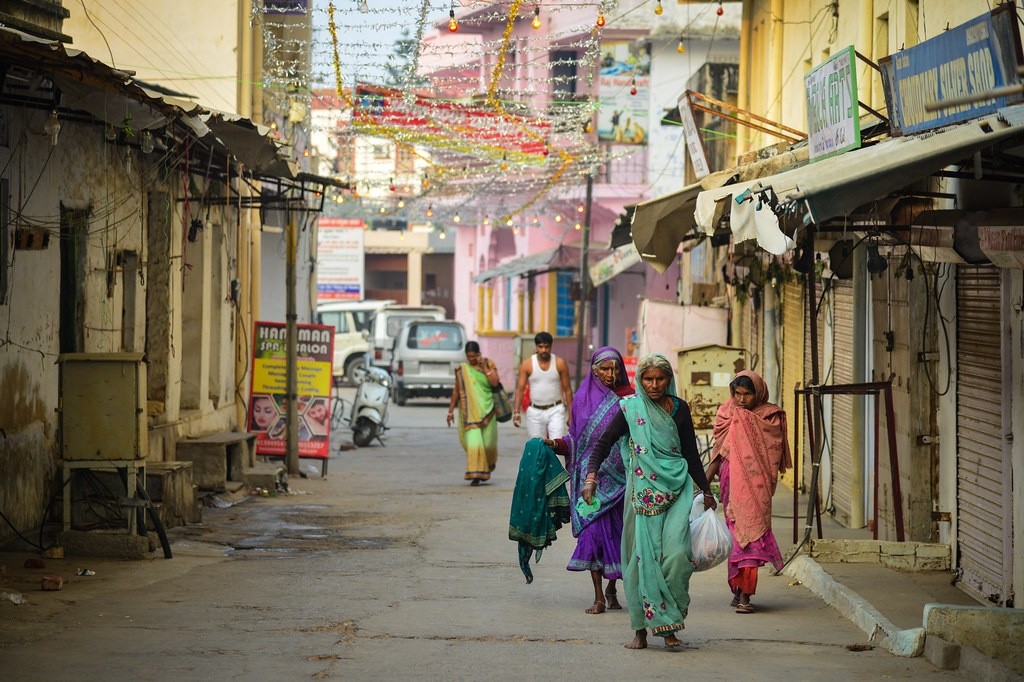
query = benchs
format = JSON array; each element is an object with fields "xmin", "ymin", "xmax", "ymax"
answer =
[{"xmin": 175, "ymin": 430, "xmax": 258, "ymax": 491}]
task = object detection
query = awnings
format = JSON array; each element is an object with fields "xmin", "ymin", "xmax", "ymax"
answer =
[{"xmin": 632, "ymin": 110, "xmax": 1024, "ymax": 273}]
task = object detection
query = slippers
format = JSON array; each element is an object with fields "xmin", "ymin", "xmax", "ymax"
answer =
[
  {"xmin": 736, "ymin": 604, "xmax": 752, "ymax": 612},
  {"xmin": 731, "ymin": 596, "xmax": 740, "ymax": 606}
]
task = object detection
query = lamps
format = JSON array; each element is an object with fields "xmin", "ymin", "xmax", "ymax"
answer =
[{"xmin": 868, "ymin": 237, "xmax": 888, "ymax": 274}]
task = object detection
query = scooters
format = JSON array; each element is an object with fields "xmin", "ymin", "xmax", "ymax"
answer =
[{"xmin": 344, "ymin": 364, "xmax": 393, "ymax": 448}]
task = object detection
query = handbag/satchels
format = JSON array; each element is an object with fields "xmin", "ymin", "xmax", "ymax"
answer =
[{"xmin": 485, "ymin": 358, "xmax": 512, "ymax": 422}]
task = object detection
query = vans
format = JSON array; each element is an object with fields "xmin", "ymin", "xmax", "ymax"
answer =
[
  {"xmin": 371, "ymin": 304, "xmax": 447, "ymax": 378},
  {"xmin": 388, "ymin": 319, "xmax": 473, "ymax": 406},
  {"xmin": 315, "ymin": 297, "xmax": 398, "ymax": 388}
]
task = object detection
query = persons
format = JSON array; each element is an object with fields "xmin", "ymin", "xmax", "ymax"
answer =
[
  {"xmin": 706, "ymin": 370, "xmax": 792, "ymax": 614},
  {"xmin": 581, "ymin": 352, "xmax": 717, "ymax": 649},
  {"xmin": 253, "ymin": 398, "xmax": 276, "ymax": 431},
  {"xmin": 513, "ymin": 332, "xmax": 573, "ymax": 470},
  {"xmin": 542, "ymin": 346, "xmax": 636, "ymax": 615},
  {"xmin": 447, "ymin": 341, "xmax": 499, "ymax": 486},
  {"xmin": 306, "ymin": 399, "xmax": 328, "ymax": 436}
]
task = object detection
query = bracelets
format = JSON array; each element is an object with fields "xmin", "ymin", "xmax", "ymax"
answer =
[
  {"xmin": 486, "ymin": 372, "xmax": 492, "ymax": 376},
  {"xmin": 448, "ymin": 413, "xmax": 453, "ymax": 416},
  {"xmin": 705, "ymin": 494, "xmax": 713, "ymax": 498},
  {"xmin": 552, "ymin": 439, "xmax": 556, "ymax": 449},
  {"xmin": 585, "ymin": 477, "xmax": 599, "ymax": 484}
]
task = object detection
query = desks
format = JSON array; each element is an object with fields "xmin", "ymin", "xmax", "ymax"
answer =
[{"xmin": 63, "ymin": 460, "xmax": 147, "ymax": 534}]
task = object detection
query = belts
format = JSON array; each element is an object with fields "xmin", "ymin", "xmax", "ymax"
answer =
[{"xmin": 530, "ymin": 400, "xmax": 561, "ymax": 410}]
